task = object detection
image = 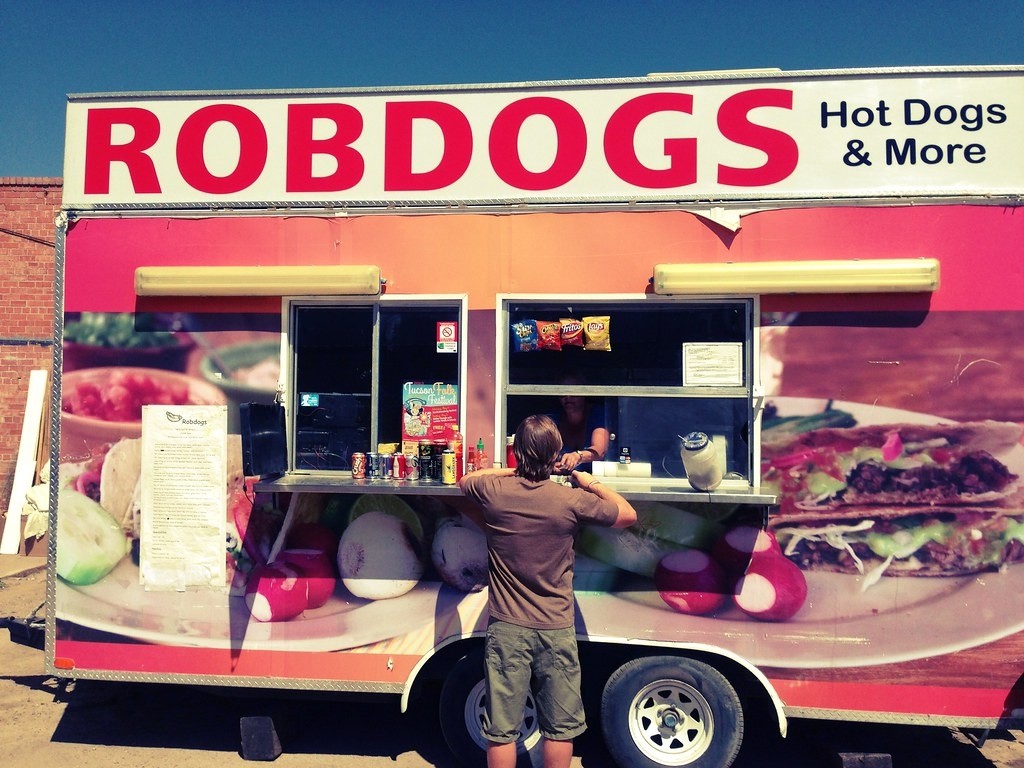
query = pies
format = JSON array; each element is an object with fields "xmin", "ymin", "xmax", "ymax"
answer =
[{"xmin": 770, "ymin": 422, "xmax": 1024, "ymax": 575}]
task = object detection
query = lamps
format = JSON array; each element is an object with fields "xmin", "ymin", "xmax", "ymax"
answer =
[
  {"xmin": 650, "ymin": 257, "xmax": 941, "ymax": 298},
  {"xmin": 133, "ymin": 265, "xmax": 387, "ymax": 299}
]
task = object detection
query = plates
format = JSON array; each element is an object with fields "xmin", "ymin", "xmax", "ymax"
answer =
[
  {"xmin": 53, "ymin": 496, "xmax": 476, "ymax": 652},
  {"xmin": 570, "ymin": 394, "xmax": 1023, "ymax": 670}
]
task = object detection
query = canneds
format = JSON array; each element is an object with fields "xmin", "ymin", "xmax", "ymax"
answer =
[{"xmin": 351, "ymin": 439, "xmax": 457, "ymax": 484}]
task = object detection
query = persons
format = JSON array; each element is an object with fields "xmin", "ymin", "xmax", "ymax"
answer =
[
  {"xmin": 457, "ymin": 412, "xmax": 637, "ymax": 768},
  {"xmin": 544, "ymin": 375, "xmax": 617, "ymax": 476}
]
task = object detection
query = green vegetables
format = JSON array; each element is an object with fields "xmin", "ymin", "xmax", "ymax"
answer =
[{"xmin": 64, "ymin": 311, "xmax": 177, "ymax": 348}]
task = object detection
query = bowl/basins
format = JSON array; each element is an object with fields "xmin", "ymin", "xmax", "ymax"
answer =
[
  {"xmin": 59, "ymin": 366, "xmax": 228, "ymax": 466},
  {"xmin": 63, "ymin": 331, "xmax": 196, "ymax": 374},
  {"xmin": 199, "ymin": 342, "xmax": 279, "ymax": 433}
]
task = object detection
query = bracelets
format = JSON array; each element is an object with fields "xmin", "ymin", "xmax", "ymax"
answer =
[
  {"xmin": 588, "ymin": 480, "xmax": 600, "ymax": 491},
  {"xmin": 576, "ymin": 450, "xmax": 585, "ymax": 463}
]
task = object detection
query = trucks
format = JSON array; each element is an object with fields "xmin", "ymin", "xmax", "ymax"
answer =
[{"xmin": 42, "ymin": 60, "xmax": 1022, "ymax": 768}]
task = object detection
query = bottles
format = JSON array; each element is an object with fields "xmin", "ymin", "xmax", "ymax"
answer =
[
  {"xmin": 681, "ymin": 432, "xmax": 722, "ymax": 492},
  {"xmin": 467, "ymin": 437, "xmax": 488, "ymax": 475},
  {"xmin": 446, "ymin": 425, "xmax": 463, "ymax": 482}
]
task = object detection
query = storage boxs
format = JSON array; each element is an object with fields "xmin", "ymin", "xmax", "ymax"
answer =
[{"xmin": 401, "ymin": 382, "xmax": 460, "ymax": 456}]
